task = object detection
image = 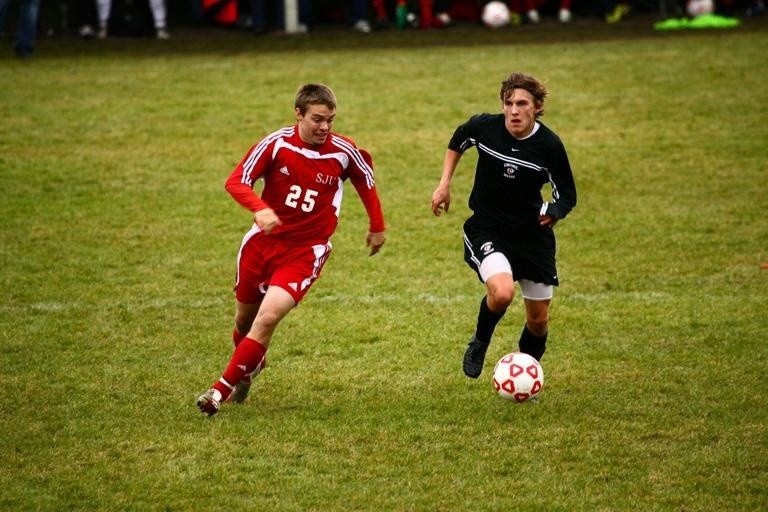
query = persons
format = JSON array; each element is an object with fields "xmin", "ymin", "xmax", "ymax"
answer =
[
  {"xmin": 1, "ymin": 1, "xmax": 633, "ymax": 54},
  {"xmin": 195, "ymin": 82, "xmax": 390, "ymax": 419},
  {"xmin": 429, "ymin": 71, "xmax": 578, "ymax": 379}
]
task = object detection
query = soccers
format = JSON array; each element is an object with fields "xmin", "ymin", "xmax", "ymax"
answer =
[
  {"xmin": 482, "ymin": 2, "xmax": 510, "ymax": 29},
  {"xmin": 493, "ymin": 352, "xmax": 543, "ymax": 401}
]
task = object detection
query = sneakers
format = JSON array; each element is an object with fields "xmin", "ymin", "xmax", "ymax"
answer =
[
  {"xmin": 462, "ymin": 334, "xmax": 490, "ymax": 379},
  {"xmin": 225, "ymin": 380, "xmax": 250, "ymax": 406},
  {"xmin": 196, "ymin": 388, "xmax": 221, "ymax": 418}
]
